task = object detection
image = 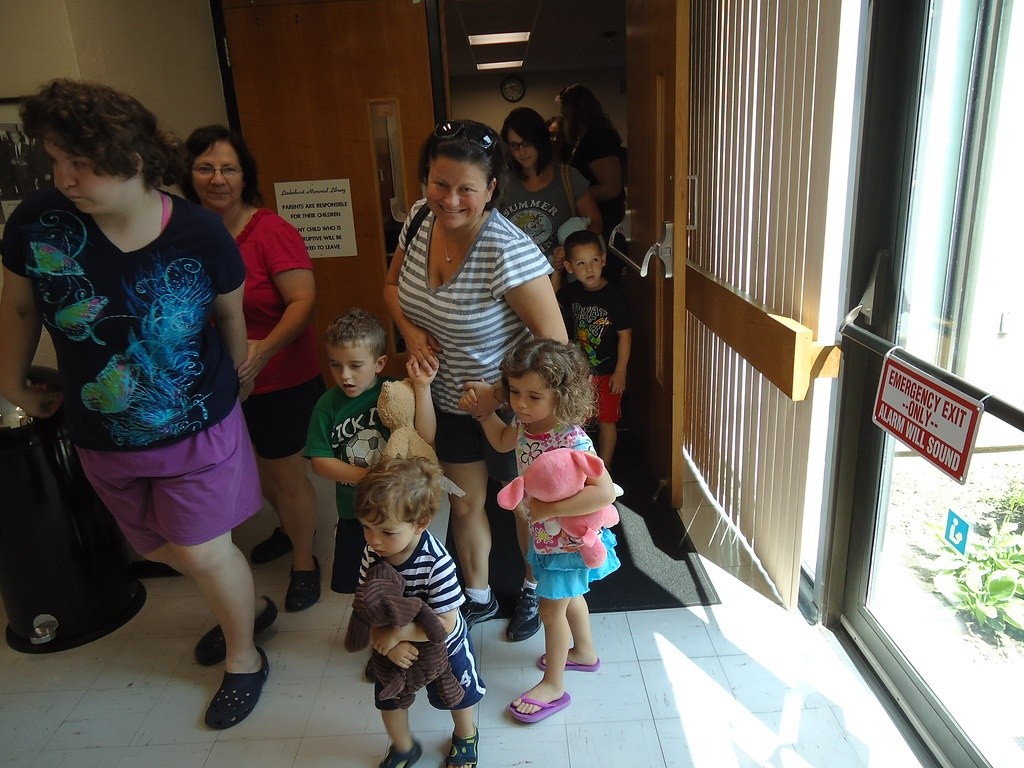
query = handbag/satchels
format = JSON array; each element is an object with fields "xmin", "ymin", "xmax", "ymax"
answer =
[{"xmin": 556, "ymin": 164, "xmax": 606, "ymax": 282}]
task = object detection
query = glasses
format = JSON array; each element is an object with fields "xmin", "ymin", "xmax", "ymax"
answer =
[
  {"xmin": 508, "ymin": 139, "xmax": 533, "ymax": 152},
  {"xmin": 434, "ymin": 120, "xmax": 498, "ymax": 150},
  {"xmin": 189, "ymin": 165, "xmax": 244, "ymax": 179}
]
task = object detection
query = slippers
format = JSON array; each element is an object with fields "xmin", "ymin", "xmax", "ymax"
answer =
[
  {"xmin": 536, "ymin": 651, "xmax": 600, "ymax": 672},
  {"xmin": 508, "ymin": 686, "xmax": 571, "ymax": 724}
]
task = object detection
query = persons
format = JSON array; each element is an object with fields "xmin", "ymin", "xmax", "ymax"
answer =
[
  {"xmin": 357, "ymin": 455, "xmax": 486, "ymax": 766},
  {"xmin": 559, "ymin": 83, "xmax": 625, "ymax": 241},
  {"xmin": 460, "ymin": 339, "xmax": 621, "ymax": 724},
  {"xmin": 500, "ymin": 107, "xmax": 603, "ymax": 271},
  {"xmin": 549, "ymin": 230, "xmax": 633, "ymax": 471},
  {"xmin": 302, "ymin": 308, "xmax": 439, "ymax": 595},
  {"xmin": 178, "ymin": 126, "xmax": 326, "ymax": 614},
  {"xmin": 383, "ymin": 120, "xmax": 568, "ymax": 641},
  {"xmin": 544, "ymin": 116, "xmax": 565, "ymax": 144},
  {"xmin": 0, "ymin": 78, "xmax": 278, "ymax": 730}
]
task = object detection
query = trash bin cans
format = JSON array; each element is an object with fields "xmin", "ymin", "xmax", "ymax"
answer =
[{"xmin": 0, "ymin": 366, "xmax": 147, "ymax": 654}]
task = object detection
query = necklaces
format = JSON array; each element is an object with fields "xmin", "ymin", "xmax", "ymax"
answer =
[{"xmin": 441, "ymin": 224, "xmax": 469, "ymax": 263}]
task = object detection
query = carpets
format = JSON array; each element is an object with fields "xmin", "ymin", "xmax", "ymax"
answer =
[{"xmin": 443, "ymin": 428, "xmax": 722, "ymax": 620}]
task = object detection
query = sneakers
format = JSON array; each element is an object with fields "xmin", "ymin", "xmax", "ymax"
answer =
[
  {"xmin": 505, "ymin": 589, "xmax": 544, "ymax": 641},
  {"xmin": 461, "ymin": 590, "xmax": 499, "ymax": 630}
]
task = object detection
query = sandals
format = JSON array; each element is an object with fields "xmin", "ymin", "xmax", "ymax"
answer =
[
  {"xmin": 205, "ymin": 646, "xmax": 267, "ymax": 731},
  {"xmin": 446, "ymin": 724, "xmax": 478, "ymax": 768},
  {"xmin": 251, "ymin": 525, "xmax": 292, "ymax": 563},
  {"xmin": 378, "ymin": 736, "xmax": 421, "ymax": 768},
  {"xmin": 195, "ymin": 595, "xmax": 278, "ymax": 665},
  {"xmin": 284, "ymin": 557, "xmax": 319, "ymax": 610}
]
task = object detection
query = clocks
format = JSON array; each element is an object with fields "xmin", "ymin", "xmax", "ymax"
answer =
[{"xmin": 500, "ymin": 76, "xmax": 526, "ymax": 102}]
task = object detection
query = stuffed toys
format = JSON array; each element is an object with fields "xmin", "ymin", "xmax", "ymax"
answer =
[
  {"xmin": 496, "ymin": 448, "xmax": 624, "ymax": 569},
  {"xmin": 556, "ymin": 216, "xmax": 591, "ymax": 244},
  {"xmin": 377, "ymin": 378, "xmax": 439, "ymax": 475},
  {"xmin": 342, "ymin": 561, "xmax": 465, "ymax": 709}
]
